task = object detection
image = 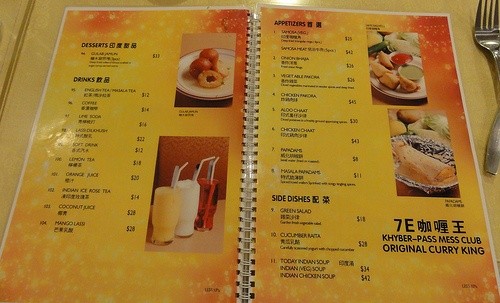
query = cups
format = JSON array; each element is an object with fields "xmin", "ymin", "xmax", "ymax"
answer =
[
  {"xmin": 174, "ymin": 180, "xmax": 200, "ymax": 238},
  {"xmin": 194, "ymin": 177, "xmax": 218, "ymax": 233},
  {"xmin": 150, "ymin": 187, "xmax": 181, "ymax": 246}
]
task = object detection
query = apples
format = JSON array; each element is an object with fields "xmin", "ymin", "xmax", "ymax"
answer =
[{"xmin": 189, "ymin": 48, "xmax": 232, "ymax": 88}]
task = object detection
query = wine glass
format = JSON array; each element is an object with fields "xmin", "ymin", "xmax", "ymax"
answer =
[{"xmin": 396, "ymin": 110, "xmax": 419, "ymax": 135}]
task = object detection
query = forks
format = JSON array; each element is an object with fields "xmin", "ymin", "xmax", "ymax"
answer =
[{"xmin": 472, "ymin": 0, "xmax": 500, "ymax": 88}]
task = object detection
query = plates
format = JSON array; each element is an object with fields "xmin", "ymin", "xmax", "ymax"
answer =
[
  {"xmin": 371, "ymin": 55, "xmax": 427, "ymax": 100},
  {"xmin": 391, "ymin": 135, "xmax": 459, "ymax": 191},
  {"xmin": 176, "ymin": 48, "xmax": 235, "ymax": 102}
]
